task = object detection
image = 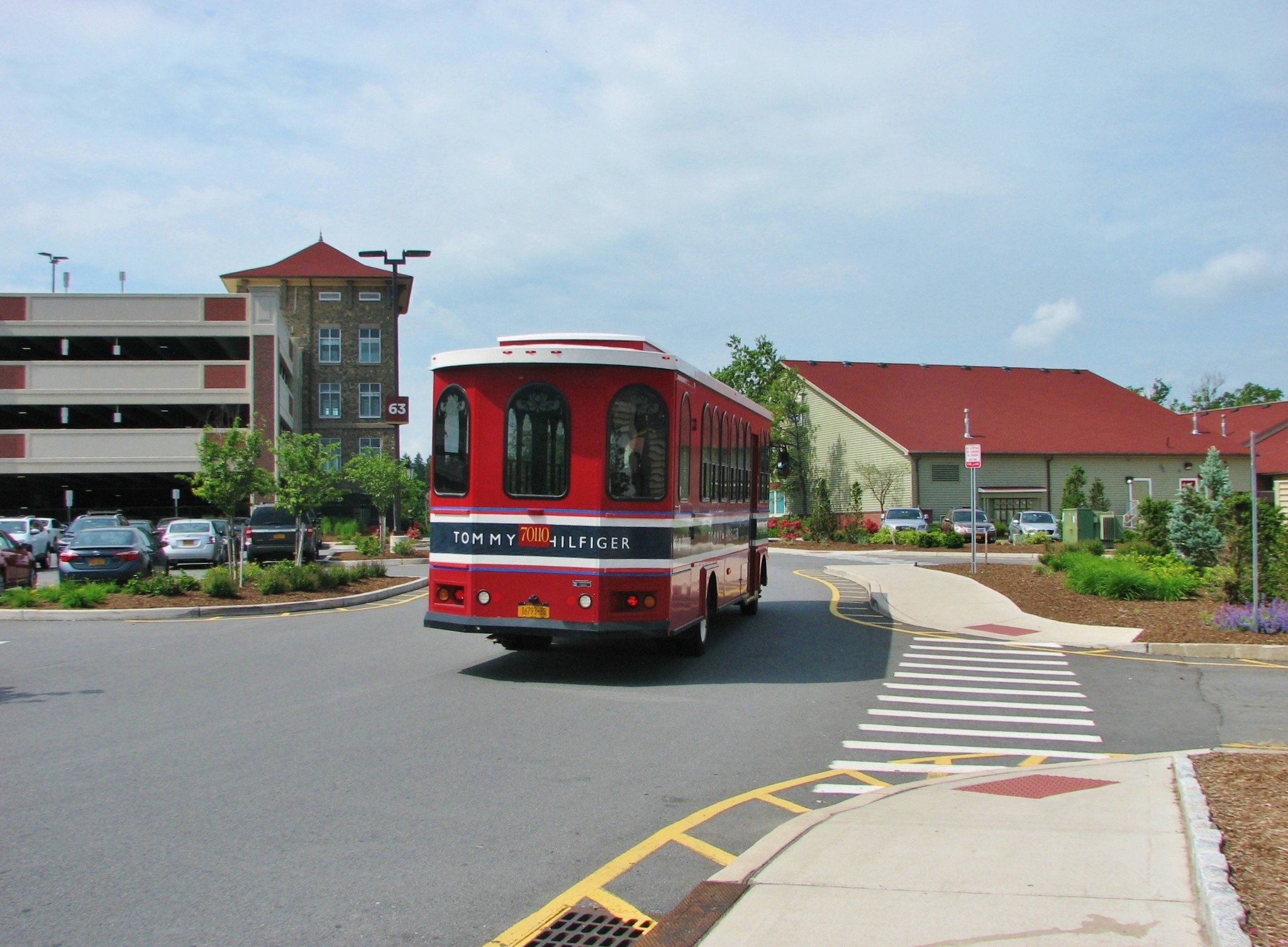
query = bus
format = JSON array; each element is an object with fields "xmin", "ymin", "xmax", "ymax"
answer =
[{"xmin": 422, "ymin": 332, "xmax": 792, "ymax": 657}]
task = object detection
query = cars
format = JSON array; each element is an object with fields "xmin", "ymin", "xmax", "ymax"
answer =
[
  {"xmin": 36, "ymin": 517, "xmax": 249, "ymax": 551},
  {"xmin": 58, "ymin": 526, "xmax": 169, "ymax": 586},
  {"xmin": 880, "ymin": 507, "xmax": 930, "ymax": 533},
  {"xmin": 940, "ymin": 508, "xmax": 996, "ymax": 544},
  {"xmin": 160, "ymin": 519, "xmax": 228, "ymax": 567},
  {"xmin": 1007, "ymin": 510, "xmax": 1061, "ymax": 544},
  {"xmin": 0, "ymin": 515, "xmax": 53, "ymax": 570},
  {"xmin": 0, "ymin": 529, "xmax": 39, "ymax": 591}
]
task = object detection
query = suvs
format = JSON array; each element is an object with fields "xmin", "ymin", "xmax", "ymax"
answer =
[
  {"xmin": 57, "ymin": 509, "xmax": 130, "ymax": 565},
  {"xmin": 245, "ymin": 500, "xmax": 324, "ymax": 565}
]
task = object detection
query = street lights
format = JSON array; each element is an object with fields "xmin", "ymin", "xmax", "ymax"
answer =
[
  {"xmin": 39, "ymin": 252, "xmax": 69, "ymax": 294},
  {"xmin": 359, "ymin": 250, "xmax": 432, "ymax": 535}
]
